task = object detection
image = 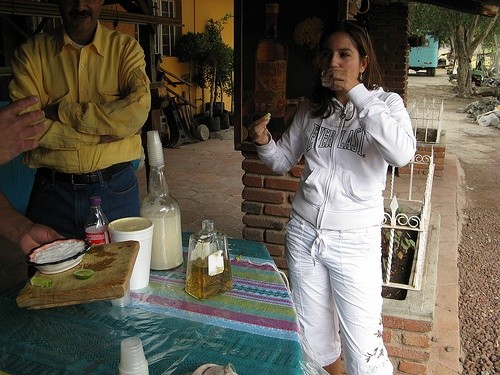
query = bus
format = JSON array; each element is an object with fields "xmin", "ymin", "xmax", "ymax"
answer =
[{"xmin": 407, "ymin": 27, "xmax": 439, "ymax": 77}]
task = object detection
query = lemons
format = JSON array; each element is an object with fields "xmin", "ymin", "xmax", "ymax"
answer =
[
  {"xmin": 31, "ymin": 276, "xmax": 52, "ymax": 287},
  {"xmin": 73, "ymin": 268, "xmax": 94, "ymax": 279}
]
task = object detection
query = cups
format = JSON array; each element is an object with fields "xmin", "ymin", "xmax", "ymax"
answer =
[
  {"xmin": 146, "ymin": 130, "xmax": 165, "ymax": 168},
  {"xmin": 321, "ymin": 70, "xmax": 333, "ymax": 87},
  {"xmin": 110, "ymin": 280, "xmax": 130, "ymax": 307},
  {"xmin": 106, "ymin": 216, "xmax": 154, "ymax": 291},
  {"xmin": 118, "ymin": 336, "xmax": 150, "ymax": 375}
]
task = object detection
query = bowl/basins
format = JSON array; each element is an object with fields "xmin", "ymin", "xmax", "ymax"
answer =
[{"xmin": 27, "ymin": 238, "xmax": 91, "ymax": 274}]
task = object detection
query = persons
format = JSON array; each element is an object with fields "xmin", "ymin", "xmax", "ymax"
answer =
[
  {"xmin": 251, "ymin": 22, "xmax": 416, "ymax": 375},
  {"xmin": 0, "ymin": 95, "xmax": 64, "ymax": 255},
  {"xmin": 8, "ymin": 0, "xmax": 151, "ymax": 278}
]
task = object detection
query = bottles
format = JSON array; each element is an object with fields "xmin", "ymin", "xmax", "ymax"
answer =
[
  {"xmin": 85, "ymin": 196, "xmax": 112, "ymax": 246},
  {"xmin": 139, "ymin": 166, "xmax": 184, "ymax": 271},
  {"xmin": 185, "ymin": 220, "xmax": 233, "ymax": 301}
]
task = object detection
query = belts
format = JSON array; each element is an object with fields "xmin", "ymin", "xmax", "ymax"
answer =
[{"xmin": 37, "ymin": 161, "xmax": 132, "ymax": 186}]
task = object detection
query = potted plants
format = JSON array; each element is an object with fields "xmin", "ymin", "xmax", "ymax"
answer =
[{"xmin": 176, "ymin": 13, "xmax": 235, "ymax": 132}]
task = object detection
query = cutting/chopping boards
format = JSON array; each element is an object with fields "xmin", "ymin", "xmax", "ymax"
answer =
[{"xmin": 16, "ymin": 240, "xmax": 140, "ymax": 308}]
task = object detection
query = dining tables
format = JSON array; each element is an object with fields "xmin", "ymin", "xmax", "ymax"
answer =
[{"xmin": 0, "ymin": 230, "xmax": 305, "ymax": 375}]
